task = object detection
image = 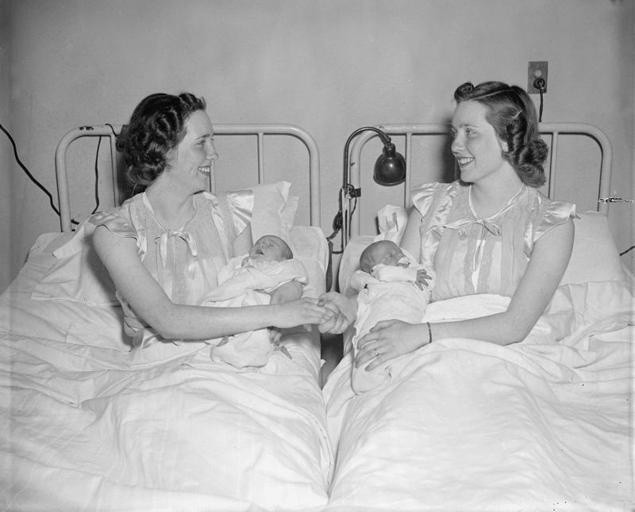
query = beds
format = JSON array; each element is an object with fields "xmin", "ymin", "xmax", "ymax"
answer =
[
  {"xmin": 0, "ymin": 124, "xmax": 324, "ymax": 512},
  {"xmin": 343, "ymin": 124, "xmax": 635, "ymax": 512}
]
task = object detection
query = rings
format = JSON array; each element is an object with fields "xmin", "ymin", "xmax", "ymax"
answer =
[{"xmin": 374, "ymin": 349, "xmax": 379, "ymax": 356}]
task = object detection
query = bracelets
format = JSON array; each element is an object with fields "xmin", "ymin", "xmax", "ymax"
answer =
[{"xmin": 427, "ymin": 322, "xmax": 432, "ymax": 344}]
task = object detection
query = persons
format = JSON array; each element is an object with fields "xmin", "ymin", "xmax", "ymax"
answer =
[
  {"xmin": 350, "ymin": 240, "xmax": 437, "ymax": 392},
  {"xmin": 201, "ymin": 234, "xmax": 309, "ymax": 367},
  {"xmin": 91, "ymin": 92, "xmax": 334, "ymax": 346},
  {"xmin": 303, "ymin": 81, "xmax": 575, "ymax": 370}
]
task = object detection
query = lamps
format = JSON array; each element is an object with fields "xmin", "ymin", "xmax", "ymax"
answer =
[{"xmin": 343, "ymin": 126, "xmax": 406, "ymax": 190}]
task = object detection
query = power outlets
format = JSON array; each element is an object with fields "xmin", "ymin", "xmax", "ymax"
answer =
[{"xmin": 528, "ymin": 61, "xmax": 547, "ymax": 93}]
